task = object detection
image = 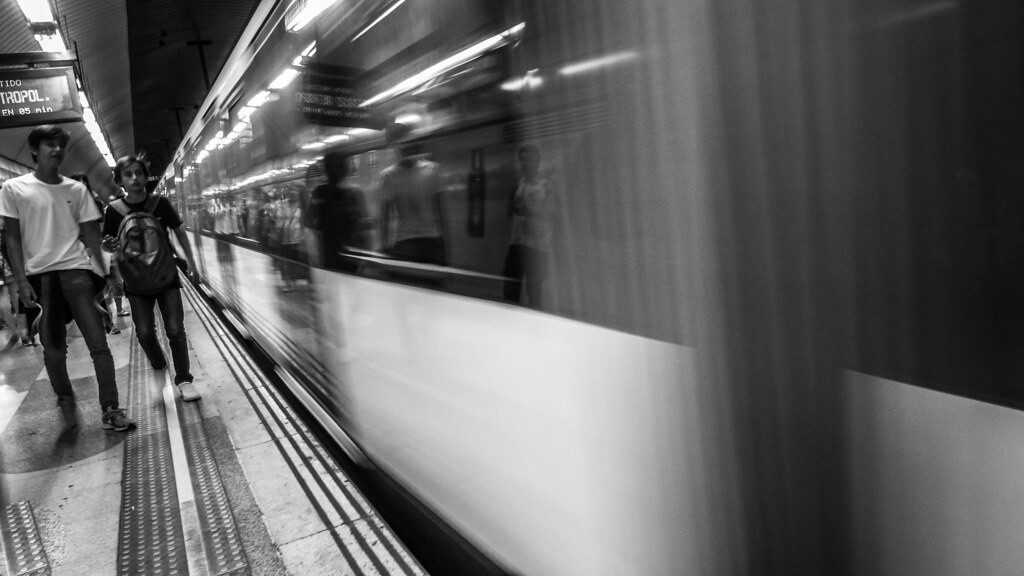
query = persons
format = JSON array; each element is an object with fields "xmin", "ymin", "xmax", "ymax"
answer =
[
  {"xmin": 306, "ymin": 149, "xmax": 378, "ymax": 347},
  {"xmin": 102, "ymin": 155, "xmax": 202, "ymax": 402},
  {"xmin": 380, "ymin": 122, "xmax": 455, "ymax": 291},
  {"xmin": 0, "ymin": 172, "xmax": 131, "ymax": 347},
  {"xmin": 0, "ymin": 125, "xmax": 137, "ymax": 432},
  {"xmin": 502, "ymin": 145, "xmax": 556, "ymax": 307},
  {"xmin": 188, "ymin": 183, "xmax": 310, "ymax": 331}
]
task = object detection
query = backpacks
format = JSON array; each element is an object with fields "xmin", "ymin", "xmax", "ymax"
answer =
[{"xmin": 108, "ymin": 193, "xmax": 177, "ymax": 296}]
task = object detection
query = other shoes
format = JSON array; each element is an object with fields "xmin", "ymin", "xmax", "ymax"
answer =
[
  {"xmin": 110, "ymin": 327, "xmax": 120, "ymax": 334},
  {"xmin": 117, "ymin": 309, "xmax": 130, "ymax": 316}
]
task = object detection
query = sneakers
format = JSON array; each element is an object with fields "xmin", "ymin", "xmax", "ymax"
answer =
[
  {"xmin": 151, "ymin": 359, "xmax": 168, "ymax": 393},
  {"xmin": 102, "ymin": 408, "xmax": 136, "ymax": 432},
  {"xmin": 59, "ymin": 400, "xmax": 78, "ymax": 427},
  {"xmin": 179, "ymin": 381, "xmax": 201, "ymax": 401}
]
